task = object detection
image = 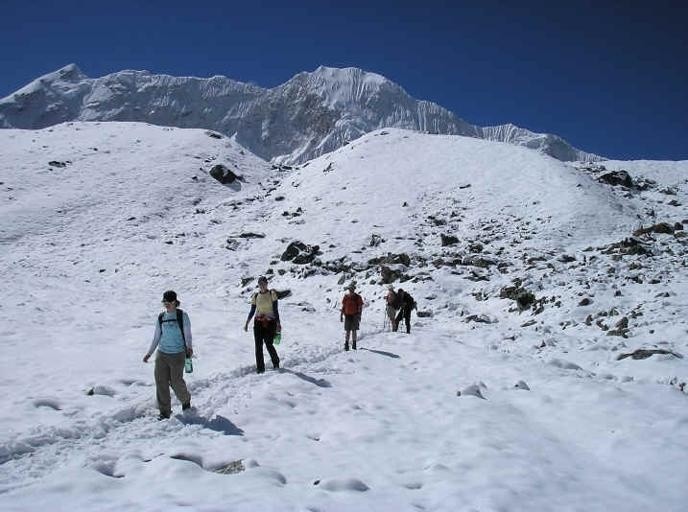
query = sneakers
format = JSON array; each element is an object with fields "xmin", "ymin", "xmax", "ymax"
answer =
[
  {"xmin": 256, "ymin": 368, "xmax": 265, "ymax": 374},
  {"xmin": 182, "ymin": 403, "xmax": 190, "ymax": 410},
  {"xmin": 274, "ymin": 363, "xmax": 280, "ymax": 368},
  {"xmin": 157, "ymin": 415, "xmax": 171, "ymax": 421}
]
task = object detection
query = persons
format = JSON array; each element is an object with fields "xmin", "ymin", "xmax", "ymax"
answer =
[
  {"xmin": 143, "ymin": 290, "xmax": 194, "ymax": 421},
  {"xmin": 243, "ymin": 275, "xmax": 283, "ymax": 375},
  {"xmin": 382, "ymin": 283, "xmax": 398, "ymax": 331},
  {"xmin": 339, "ymin": 284, "xmax": 365, "ymax": 351},
  {"xmin": 394, "ymin": 289, "xmax": 414, "ymax": 333}
]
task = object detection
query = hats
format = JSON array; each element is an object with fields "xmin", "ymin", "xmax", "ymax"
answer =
[{"xmin": 161, "ymin": 290, "xmax": 180, "ymax": 307}]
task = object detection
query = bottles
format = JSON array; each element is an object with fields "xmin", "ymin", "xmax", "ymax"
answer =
[
  {"xmin": 185, "ymin": 353, "xmax": 193, "ymax": 373},
  {"xmin": 275, "ymin": 332, "xmax": 281, "ymax": 344}
]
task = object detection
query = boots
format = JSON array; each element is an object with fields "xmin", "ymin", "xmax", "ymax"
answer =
[
  {"xmin": 345, "ymin": 341, "xmax": 349, "ymax": 351},
  {"xmin": 352, "ymin": 340, "xmax": 356, "ymax": 349}
]
